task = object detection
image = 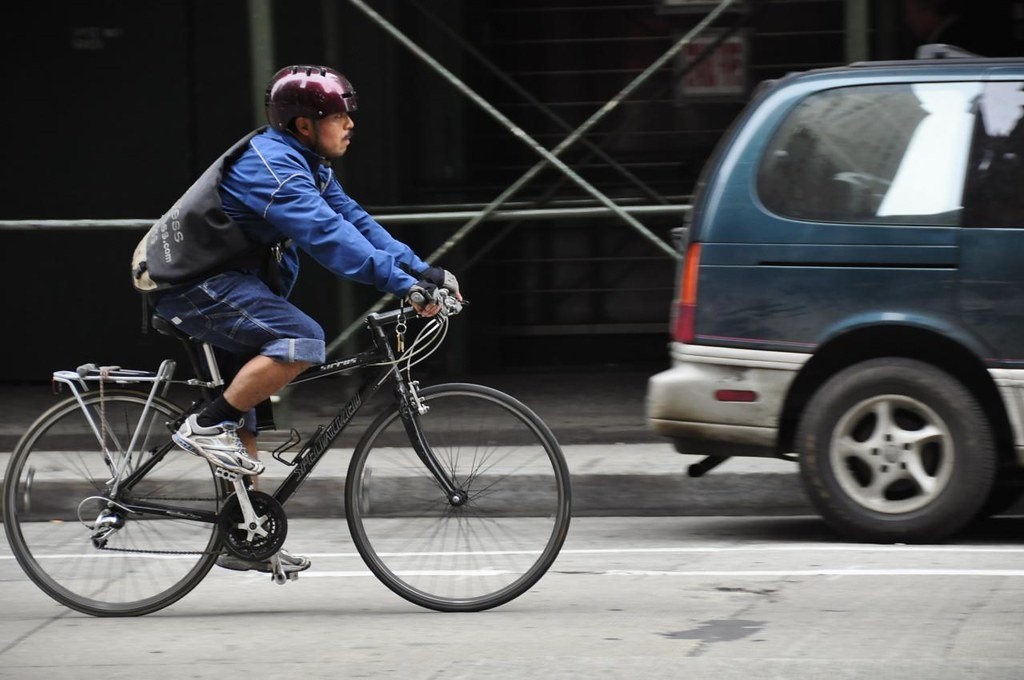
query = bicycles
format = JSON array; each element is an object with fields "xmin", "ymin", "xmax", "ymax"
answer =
[{"xmin": 0, "ymin": 287, "xmax": 572, "ymax": 618}]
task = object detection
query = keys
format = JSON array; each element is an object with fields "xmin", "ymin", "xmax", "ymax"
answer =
[{"xmin": 396, "ymin": 312, "xmax": 406, "ymax": 356}]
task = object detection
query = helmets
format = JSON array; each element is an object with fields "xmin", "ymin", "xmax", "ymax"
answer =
[{"xmin": 264, "ymin": 64, "xmax": 358, "ymax": 134}]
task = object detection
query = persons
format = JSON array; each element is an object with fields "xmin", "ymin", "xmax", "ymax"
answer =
[{"xmin": 132, "ymin": 66, "xmax": 463, "ymax": 573}]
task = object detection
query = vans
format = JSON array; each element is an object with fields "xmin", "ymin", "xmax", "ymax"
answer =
[{"xmin": 647, "ymin": 57, "xmax": 1024, "ymax": 543}]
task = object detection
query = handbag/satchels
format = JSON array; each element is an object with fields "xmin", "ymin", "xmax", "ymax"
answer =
[{"xmin": 131, "ymin": 124, "xmax": 322, "ymax": 293}]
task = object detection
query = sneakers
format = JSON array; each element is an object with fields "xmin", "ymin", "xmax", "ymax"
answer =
[
  {"xmin": 215, "ymin": 545, "xmax": 310, "ymax": 573},
  {"xmin": 171, "ymin": 413, "xmax": 265, "ymax": 476}
]
